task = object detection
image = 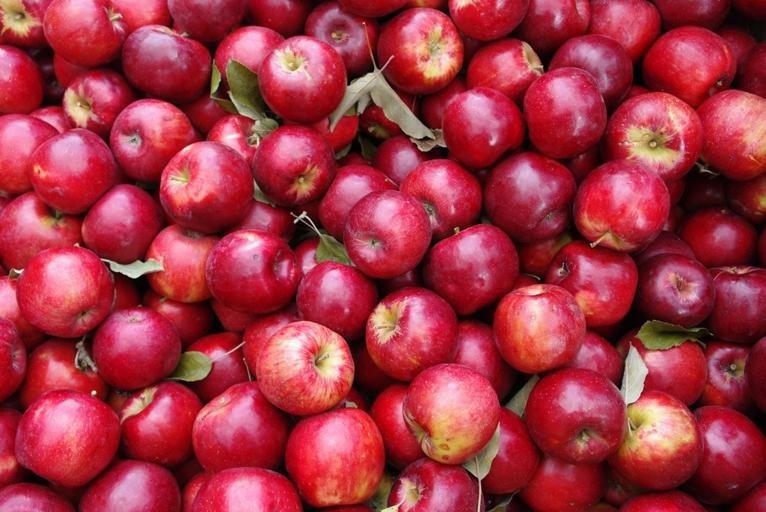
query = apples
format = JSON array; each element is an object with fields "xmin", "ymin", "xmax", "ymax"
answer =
[{"xmin": 1, "ymin": 0, "xmax": 766, "ymax": 512}]
